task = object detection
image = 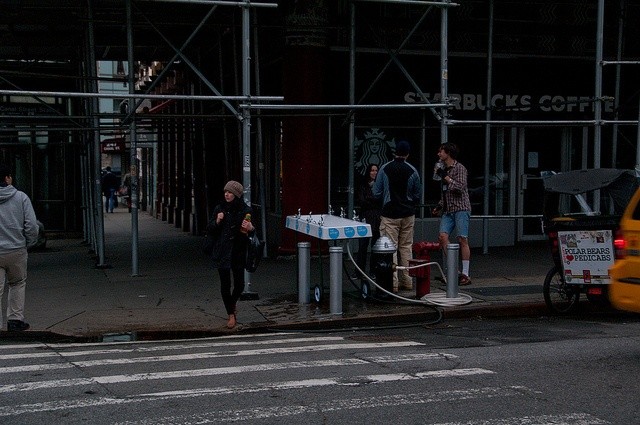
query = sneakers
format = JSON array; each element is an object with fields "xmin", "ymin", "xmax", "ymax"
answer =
[
  {"xmin": 7, "ymin": 320, "xmax": 29, "ymax": 331},
  {"xmin": 459, "ymin": 274, "xmax": 471, "ymax": 285},
  {"xmin": 351, "ymin": 271, "xmax": 364, "ymax": 280},
  {"xmin": 399, "ymin": 282, "xmax": 412, "ymax": 290}
]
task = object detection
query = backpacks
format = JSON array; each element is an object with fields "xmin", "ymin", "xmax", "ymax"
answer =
[{"xmin": 203, "ymin": 203, "xmax": 226, "ymax": 256}]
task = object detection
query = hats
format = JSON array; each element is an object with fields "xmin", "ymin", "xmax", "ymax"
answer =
[
  {"xmin": 224, "ymin": 180, "xmax": 244, "ymax": 199},
  {"xmin": 396, "ymin": 141, "xmax": 410, "ymax": 156}
]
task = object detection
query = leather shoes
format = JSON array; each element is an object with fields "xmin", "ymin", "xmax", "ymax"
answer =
[{"xmin": 227, "ymin": 314, "xmax": 236, "ymax": 329}]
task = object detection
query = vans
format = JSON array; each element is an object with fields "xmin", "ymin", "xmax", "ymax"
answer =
[{"xmin": 609, "ymin": 183, "xmax": 640, "ymax": 313}]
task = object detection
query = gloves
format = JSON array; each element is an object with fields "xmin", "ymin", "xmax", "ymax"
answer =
[
  {"xmin": 436, "ymin": 204, "xmax": 442, "ymax": 214},
  {"xmin": 435, "ymin": 167, "xmax": 449, "ymax": 179}
]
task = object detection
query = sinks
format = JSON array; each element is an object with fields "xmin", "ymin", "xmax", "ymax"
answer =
[{"xmin": 284, "ymin": 213, "xmax": 373, "ymax": 241}]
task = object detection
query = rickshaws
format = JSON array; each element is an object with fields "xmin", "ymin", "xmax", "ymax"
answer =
[{"xmin": 540, "ymin": 167, "xmax": 640, "ymax": 314}]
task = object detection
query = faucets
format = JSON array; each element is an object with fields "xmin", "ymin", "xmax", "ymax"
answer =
[
  {"xmin": 318, "ymin": 215, "xmax": 325, "ymax": 227},
  {"xmin": 327, "ymin": 204, "xmax": 335, "ymax": 215},
  {"xmin": 306, "ymin": 211, "xmax": 314, "ymax": 223},
  {"xmin": 339, "ymin": 206, "xmax": 347, "ymax": 218},
  {"xmin": 351, "ymin": 209, "xmax": 360, "ymax": 221},
  {"xmin": 296, "ymin": 208, "xmax": 303, "ymax": 219}
]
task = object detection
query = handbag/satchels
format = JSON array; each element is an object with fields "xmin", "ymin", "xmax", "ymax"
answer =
[
  {"xmin": 246, "ymin": 240, "xmax": 265, "ymax": 273},
  {"xmin": 121, "ymin": 186, "xmax": 128, "ymax": 194}
]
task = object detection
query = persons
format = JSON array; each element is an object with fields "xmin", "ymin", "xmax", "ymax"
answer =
[
  {"xmin": 0, "ymin": 167, "xmax": 40, "ymax": 332},
  {"xmin": 433, "ymin": 142, "xmax": 472, "ymax": 285},
  {"xmin": 123, "ymin": 172, "xmax": 131, "ymax": 212},
  {"xmin": 371, "ymin": 141, "xmax": 422, "ymax": 292},
  {"xmin": 101, "ymin": 166, "xmax": 120, "ymax": 213},
  {"xmin": 351, "ymin": 163, "xmax": 384, "ymax": 279},
  {"xmin": 207, "ymin": 180, "xmax": 257, "ymax": 328}
]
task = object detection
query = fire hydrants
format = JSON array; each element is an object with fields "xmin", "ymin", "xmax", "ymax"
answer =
[
  {"xmin": 371, "ymin": 235, "xmax": 396, "ymax": 306},
  {"xmin": 407, "ymin": 241, "xmax": 439, "ymax": 299}
]
